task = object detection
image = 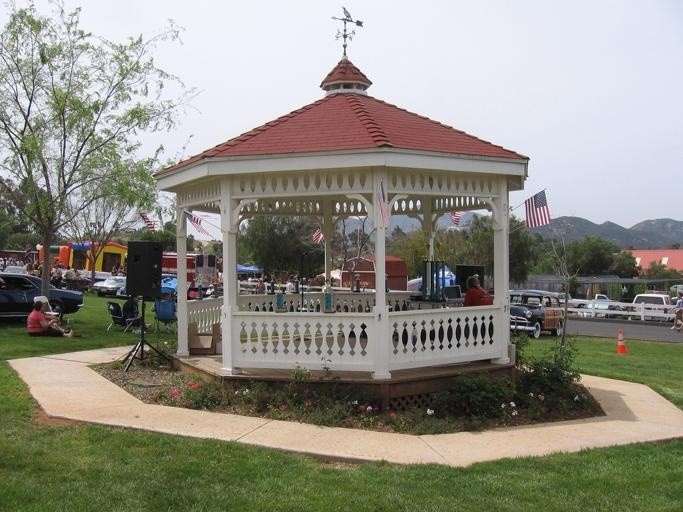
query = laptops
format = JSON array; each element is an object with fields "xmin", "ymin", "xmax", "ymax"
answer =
[{"xmin": 442, "ymin": 285, "xmax": 464, "ymax": 301}]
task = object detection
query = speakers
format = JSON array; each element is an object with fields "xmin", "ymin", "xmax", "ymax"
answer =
[
  {"xmin": 126, "ymin": 240, "xmax": 164, "ymax": 297},
  {"xmin": 456, "ymin": 264, "xmax": 485, "ymax": 293}
]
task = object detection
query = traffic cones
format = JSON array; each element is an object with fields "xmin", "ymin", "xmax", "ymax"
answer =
[{"xmin": 616, "ymin": 328, "xmax": 628, "ymax": 355}]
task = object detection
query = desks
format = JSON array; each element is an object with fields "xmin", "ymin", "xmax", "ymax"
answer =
[{"xmin": 402, "ymin": 298, "xmax": 466, "ymax": 311}]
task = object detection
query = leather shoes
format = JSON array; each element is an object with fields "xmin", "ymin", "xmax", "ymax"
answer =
[{"xmin": 66, "ymin": 327, "xmax": 73, "ymax": 338}]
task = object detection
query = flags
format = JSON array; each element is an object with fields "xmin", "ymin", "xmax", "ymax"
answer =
[
  {"xmin": 524, "ymin": 190, "xmax": 552, "ymax": 229},
  {"xmin": 139, "ymin": 211, "xmax": 155, "ymax": 235},
  {"xmin": 312, "ymin": 229, "xmax": 324, "ymax": 245},
  {"xmin": 448, "ymin": 211, "xmax": 466, "ymax": 226},
  {"xmin": 183, "ymin": 210, "xmax": 214, "ymax": 239}
]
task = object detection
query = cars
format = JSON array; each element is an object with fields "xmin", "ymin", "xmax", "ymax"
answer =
[
  {"xmin": 0, "ymin": 250, "xmax": 217, "ymax": 300},
  {"xmin": 669, "ymin": 285, "xmax": 682, "ymax": 294},
  {"xmin": 508, "ymin": 290, "xmax": 565, "ymax": 338},
  {"xmin": 0, "ymin": 273, "xmax": 83, "ymax": 322}
]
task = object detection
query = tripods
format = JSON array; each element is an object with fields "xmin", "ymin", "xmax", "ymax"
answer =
[{"xmin": 121, "ymin": 296, "xmax": 171, "ymax": 371}]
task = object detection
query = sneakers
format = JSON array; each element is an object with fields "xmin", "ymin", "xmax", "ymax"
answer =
[{"xmin": 131, "ymin": 323, "xmax": 172, "ymax": 335}]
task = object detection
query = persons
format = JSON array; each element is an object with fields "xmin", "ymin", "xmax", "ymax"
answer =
[
  {"xmin": 206, "ymin": 284, "xmax": 214, "ymax": 296},
  {"xmin": 151, "ymin": 294, "xmax": 177, "ymax": 333},
  {"xmin": 122, "ymin": 294, "xmax": 152, "ymax": 331},
  {"xmin": 111, "ymin": 263, "xmax": 128, "ymax": 276},
  {"xmin": 27, "ymin": 301, "xmax": 73, "ymax": 338},
  {"xmin": 255, "ymin": 280, "xmax": 266, "ymax": 294},
  {"xmin": 464, "ymin": 276, "xmax": 493, "ymax": 307},
  {"xmin": 0, "ymin": 248, "xmax": 81, "ymax": 290},
  {"xmin": 670, "ymin": 290, "xmax": 683, "ymax": 331}
]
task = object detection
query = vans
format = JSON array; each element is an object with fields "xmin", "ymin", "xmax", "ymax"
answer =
[{"xmin": 629, "ymin": 293, "xmax": 676, "ymax": 321}]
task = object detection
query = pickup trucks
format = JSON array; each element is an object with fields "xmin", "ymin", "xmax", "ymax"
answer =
[{"xmin": 571, "ymin": 293, "xmax": 618, "ymax": 310}]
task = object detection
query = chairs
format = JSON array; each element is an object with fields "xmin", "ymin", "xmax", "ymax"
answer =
[
  {"xmin": 154, "ymin": 298, "xmax": 177, "ymax": 333},
  {"xmin": 34, "ymin": 296, "xmax": 61, "ymax": 330},
  {"xmin": 107, "ymin": 301, "xmax": 144, "ymax": 333}
]
